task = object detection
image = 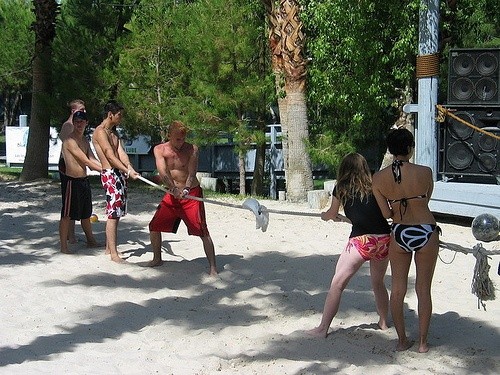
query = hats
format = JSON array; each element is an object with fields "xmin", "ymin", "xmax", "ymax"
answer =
[{"xmin": 72, "ymin": 111, "xmax": 87, "ymax": 120}]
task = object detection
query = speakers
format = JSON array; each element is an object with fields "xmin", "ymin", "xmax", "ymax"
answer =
[
  {"xmin": 446, "ymin": 48, "xmax": 500, "ymax": 105},
  {"xmin": 442, "ymin": 104, "xmax": 500, "ymax": 181}
]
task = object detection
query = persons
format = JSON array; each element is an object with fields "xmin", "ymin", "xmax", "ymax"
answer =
[
  {"xmin": 92, "ymin": 100, "xmax": 139, "ymax": 263},
  {"xmin": 304, "ymin": 153, "xmax": 391, "ymax": 338},
  {"xmin": 141, "ymin": 121, "xmax": 219, "ymax": 275},
  {"xmin": 371, "ymin": 128, "xmax": 443, "ymax": 353},
  {"xmin": 58, "ymin": 99, "xmax": 102, "ymax": 253}
]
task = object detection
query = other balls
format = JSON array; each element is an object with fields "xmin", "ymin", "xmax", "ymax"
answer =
[
  {"xmin": 471, "ymin": 213, "xmax": 500, "ymax": 241},
  {"xmin": 90, "ymin": 214, "xmax": 98, "ymax": 223}
]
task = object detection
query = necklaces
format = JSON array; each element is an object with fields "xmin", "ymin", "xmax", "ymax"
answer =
[{"xmin": 104, "ymin": 123, "xmax": 113, "ymax": 132}]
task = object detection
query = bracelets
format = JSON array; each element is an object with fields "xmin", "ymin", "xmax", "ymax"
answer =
[
  {"xmin": 170, "ymin": 186, "xmax": 178, "ymax": 192},
  {"xmin": 126, "ymin": 162, "xmax": 133, "ymax": 167},
  {"xmin": 124, "ymin": 170, "xmax": 130, "ymax": 178},
  {"xmin": 182, "ymin": 187, "xmax": 190, "ymax": 195}
]
